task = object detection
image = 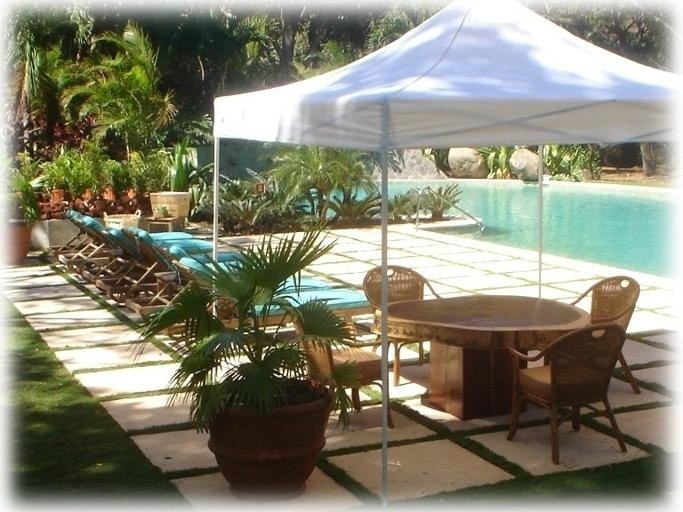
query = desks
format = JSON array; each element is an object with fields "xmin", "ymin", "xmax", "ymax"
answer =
[{"xmin": 373, "ymin": 294, "xmax": 590, "ymax": 420}]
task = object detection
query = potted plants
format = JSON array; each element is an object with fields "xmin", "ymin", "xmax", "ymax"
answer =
[
  {"xmin": 2, "ymin": 132, "xmax": 192, "ymax": 268},
  {"xmin": 125, "ymin": 211, "xmax": 367, "ymax": 502}
]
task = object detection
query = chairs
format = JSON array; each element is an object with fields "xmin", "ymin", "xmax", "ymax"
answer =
[
  {"xmin": 50, "ymin": 205, "xmax": 368, "ymax": 345},
  {"xmin": 545, "ymin": 276, "xmax": 641, "ymax": 396},
  {"xmin": 291, "ymin": 319, "xmax": 393, "ymax": 429},
  {"xmin": 507, "ymin": 323, "xmax": 626, "ymax": 465},
  {"xmin": 364, "ymin": 264, "xmax": 441, "ymax": 386}
]
